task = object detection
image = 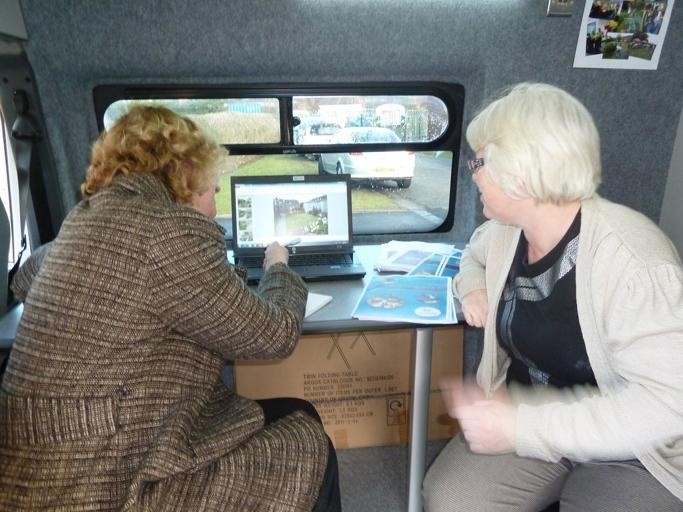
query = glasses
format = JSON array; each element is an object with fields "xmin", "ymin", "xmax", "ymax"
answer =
[{"xmin": 462, "ymin": 158, "xmax": 490, "ymax": 172}]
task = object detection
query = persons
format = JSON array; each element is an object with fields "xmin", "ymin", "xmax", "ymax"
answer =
[
  {"xmin": 586, "ymin": 3, "xmax": 663, "ymax": 59},
  {"xmin": 0, "ymin": 105, "xmax": 341, "ymax": 511},
  {"xmin": 419, "ymin": 83, "xmax": 682, "ymax": 511}
]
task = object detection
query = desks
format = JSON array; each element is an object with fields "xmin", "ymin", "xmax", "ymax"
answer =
[{"xmin": 1, "ymin": 245, "xmax": 465, "ymax": 511}]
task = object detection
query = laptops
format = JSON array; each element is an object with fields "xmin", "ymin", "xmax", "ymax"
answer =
[{"xmin": 230, "ymin": 173, "xmax": 366, "ymax": 286}]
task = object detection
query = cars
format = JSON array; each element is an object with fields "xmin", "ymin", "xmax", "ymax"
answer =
[
  {"xmin": 293, "ymin": 122, "xmax": 352, "ymax": 161},
  {"xmin": 318, "ymin": 126, "xmax": 415, "ymax": 188}
]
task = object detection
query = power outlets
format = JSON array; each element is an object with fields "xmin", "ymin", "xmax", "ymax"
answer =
[{"xmin": 546, "ymin": 0, "xmax": 574, "ymax": 17}]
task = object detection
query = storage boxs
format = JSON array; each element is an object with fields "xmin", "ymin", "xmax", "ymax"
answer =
[{"xmin": 235, "ymin": 325, "xmax": 466, "ymax": 450}]
